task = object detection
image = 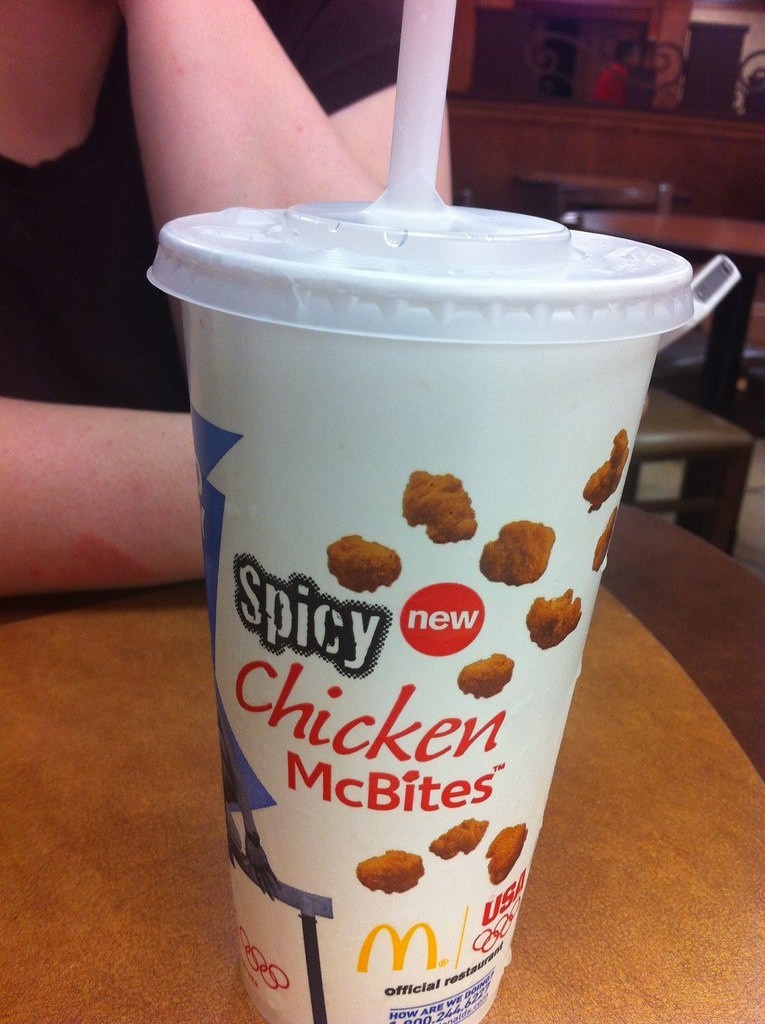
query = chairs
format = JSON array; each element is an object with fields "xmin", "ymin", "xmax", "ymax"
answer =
[
  {"xmin": 619, "ymin": 386, "xmax": 757, "ymax": 557},
  {"xmin": 549, "ymin": 173, "xmax": 764, "ymax": 440}
]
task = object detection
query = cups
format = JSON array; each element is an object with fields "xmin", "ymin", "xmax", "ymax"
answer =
[{"xmin": 146, "ymin": 192, "xmax": 693, "ymax": 1024}]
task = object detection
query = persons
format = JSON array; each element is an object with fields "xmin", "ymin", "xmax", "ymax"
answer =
[
  {"xmin": 598, "ymin": 39, "xmax": 640, "ymax": 104},
  {"xmin": 0, "ymin": 0, "xmax": 451, "ymax": 593}
]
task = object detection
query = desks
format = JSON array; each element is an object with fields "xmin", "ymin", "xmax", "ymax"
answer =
[
  {"xmin": 561, "ymin": 207, "xmax": 764, "ymax": 535},
  {"xmin": 0, "ymin": 501, "xmax": 765, "ymax": 1024},
  {"xmin": 512, "ymin": 168, "xmax": 693, "ymax": 206}
]
